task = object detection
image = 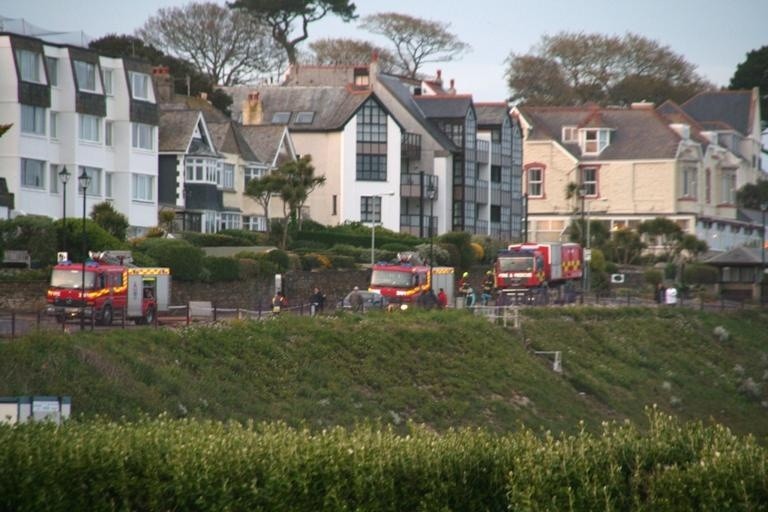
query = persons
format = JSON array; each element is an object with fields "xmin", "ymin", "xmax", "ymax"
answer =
[
  {"xmin": 436, "ymin": 288, "xmax": 448, "ymax": 310},
  {"xmin": 271, "ymin": 291, "xmax": 285, "ymax": 317},
  {"xmin": 349, "ymin": 286, "xmax": 362, "ymax": 313},
  {"xmin": 144, "ymin": 288, "xmax": 154, "ymax": 300},
  {"xmin": 309, "ymin": 286, "xmax": 322, "ymax": 315},
  {"xmin": 654, "ymin": 283, "xmax": 679, "ymax": 305},
  {"xmin": 457, "ymin": 270, "xmax": 495, "ymax": 309}
]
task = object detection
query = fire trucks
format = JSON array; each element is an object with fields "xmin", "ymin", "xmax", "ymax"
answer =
[
  {"xmin": 46, "ymin": 251, "xmax": 171, "ymax": 326},
  {"xmin": 493, "ymin": 242, "xmax": 583, "ymax": 302},
  {"xmin": 369, "ymin": 252, "xmax": 454, "ymax": 306}
]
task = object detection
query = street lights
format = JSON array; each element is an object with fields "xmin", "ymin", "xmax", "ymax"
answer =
[
  {"xmin": 426, "ymin": 181, "xmax": 437, "ymax": 266},
  {"xmin": 577, "ymin": 185, "xmax": 588, "ymax": 295},
  {"xmin": 59, "ymin": 164, "xmax": 92, "ymax": 327}
]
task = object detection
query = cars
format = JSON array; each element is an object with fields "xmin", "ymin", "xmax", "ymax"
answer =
[{"xmin": 337, "ymin": 291, "xmax": 387, "ymax": 309}]
